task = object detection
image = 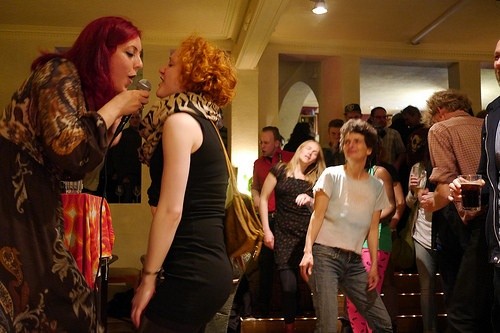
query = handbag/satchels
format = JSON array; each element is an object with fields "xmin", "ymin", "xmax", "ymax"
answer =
[{"xmin": 223, "ymin": 193, "xmax": 265, "ymax": 279}]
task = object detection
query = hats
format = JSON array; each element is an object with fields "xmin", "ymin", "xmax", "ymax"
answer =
[{"xmin": 345, "ymin": 104, "xmax": 361, "ymax": 115}]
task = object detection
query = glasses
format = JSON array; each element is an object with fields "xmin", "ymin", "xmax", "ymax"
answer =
[
  {"xmin": 373, "ymin": 115, "xmax": 388, "ymax": 120},
  {"xmin": 429, "ymin": 105, "xmax": 442, "ymax": 126}
]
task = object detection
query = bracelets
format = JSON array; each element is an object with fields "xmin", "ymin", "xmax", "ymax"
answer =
[{"xmin": 139, "ymin": 254, "xmax": 162, "ymax": 276}]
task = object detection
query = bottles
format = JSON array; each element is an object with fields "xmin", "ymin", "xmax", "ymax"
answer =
[{"xmin": 278, "ymin": 153, "xmax": 283, "ymax": 163}]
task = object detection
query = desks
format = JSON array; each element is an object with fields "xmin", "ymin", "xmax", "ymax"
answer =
[{"xmin": 58, "ymin": 194, "xmax": 113, "ymax": 292}]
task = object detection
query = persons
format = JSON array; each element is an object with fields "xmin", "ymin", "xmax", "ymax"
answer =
[
  {"xmin": 129, "ymin": 34, "xmax": 234, "ymax": 333},
  {"xmin": 299, "ymin": 118, "xmax": 394, "ymax": 333},
  {"xmin": 253, "ymin": 121, "xmax": 326, "ymax": 333},
  {"xmin": 321, "ymin": 89, "xmax": 486, "ymax": 333},
  {"xmin": 447, "ymin": 39, "xmax": 500, "ymax": 333},
  {"xmin": 0, "ymin": 16, "xmax": 150, "ymax": 333}
]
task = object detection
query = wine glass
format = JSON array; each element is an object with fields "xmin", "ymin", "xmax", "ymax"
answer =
[{"xmin": 412, "ymin": 163, "xmax": 427, "ymax": 201}]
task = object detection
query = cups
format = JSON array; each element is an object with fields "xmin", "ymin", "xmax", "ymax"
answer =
[
  {"xmin": 65, "ymin": 180, "xmax": 82, "ymax": 193},
  {"xmin": 459, "ymin": 174, "xmax": 482, "ymax": 212}
]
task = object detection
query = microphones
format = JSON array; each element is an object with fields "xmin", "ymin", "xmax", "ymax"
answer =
[{"xmin": 115, "ymin": 79, "xmax": 151, "ymax": 136}]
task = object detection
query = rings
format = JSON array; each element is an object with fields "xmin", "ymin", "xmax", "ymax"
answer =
[{"xmin": 140, "ymin": 106, "xmax": 144, "ymax": 110}]
